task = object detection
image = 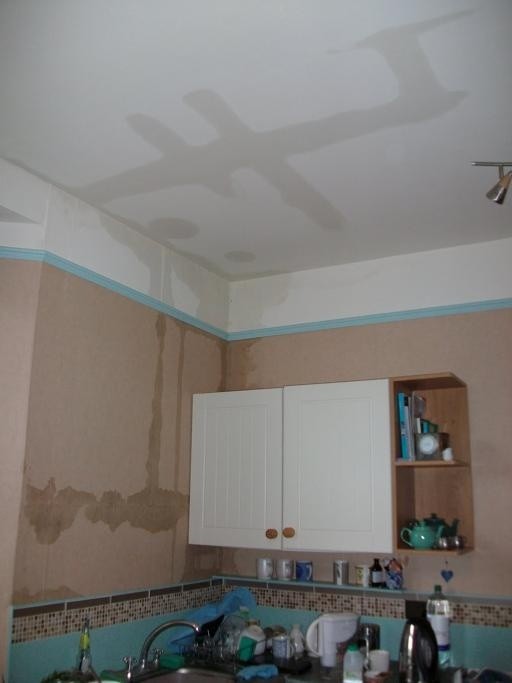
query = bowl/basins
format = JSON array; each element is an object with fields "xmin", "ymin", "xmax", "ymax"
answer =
[{"xmin": 237, "ymin": 624, "xmax": 266, "ymax": 657}]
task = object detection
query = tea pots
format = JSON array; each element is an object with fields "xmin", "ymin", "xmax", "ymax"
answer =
[{"xmin": 401, "ymin": 519, "xmax": 445, "ymax": 549}]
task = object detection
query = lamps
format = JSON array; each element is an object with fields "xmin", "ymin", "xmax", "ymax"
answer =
[{"xmin": 469, "ymin": 160, "xmax": 512, "ymax": 205}]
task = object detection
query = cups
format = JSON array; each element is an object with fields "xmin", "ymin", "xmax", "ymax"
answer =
[
  {"xmin": 364, "ymin": 650, "xmax": 390, "ymax": 673},
  {"xmin": 335, "ymin": 560, "xmax": 346, "ymax": 585},
  {"xmin": 272, "ymin": 636, "xmax": 293, "ymax": 658},
  {"xmin": 451, "ymin": 536, "xmax": 462, "ymax": 549},
  {"xmin": 437, "ymin": 536, "xmax": 449, "ymax": 548},
  {"xmin": 355, "ymin": 565, "xmax": 369, "ymax": 588},
  {"xmin": 257, "ymin": 558, "xmax": 313, "ymax": 582},
  {"xmin": 238, "ymin": 636, "xmax": 258, "ymax": 663}
]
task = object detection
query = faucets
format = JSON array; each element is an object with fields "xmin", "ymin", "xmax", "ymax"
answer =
[{"xmin": 138, "ymin": 618, "xmax": 206, "ymax": 671}]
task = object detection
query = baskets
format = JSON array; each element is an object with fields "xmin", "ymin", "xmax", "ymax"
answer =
[{"xmin": 170, "ymin": 632, "xmax": 288, "ymax": 675}]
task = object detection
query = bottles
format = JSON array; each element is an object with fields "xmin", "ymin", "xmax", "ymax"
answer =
[
  {"xmin": 78, "ymin": 617, "xmax": 93, "ymax": 671},
  {"xmin": 361, "ymin": 624, "xmax": 379, "ymax": 651},
  {"xmin": 370, "ymin": 558, "xmax": 382, "ymax": 588},
  {"xmin": 290, "ymin": 624, "xmax": 305, "ymax": 652},
  {"xmin": 427, "ymin": 585, "xmax": 451, "ymax": 674},
  {"xmin": 343, "ymin": 644, "xmax": 364, "ymax": 683}
]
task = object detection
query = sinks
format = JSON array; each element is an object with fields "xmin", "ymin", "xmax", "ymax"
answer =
[{"xmin": 137, "ymin": 664, "xmax": 236, "ymax": 683}]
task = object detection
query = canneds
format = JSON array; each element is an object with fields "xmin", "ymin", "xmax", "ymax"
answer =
[{"xmin": 333, "ymin": 560, "xmax": 349, "ymax": 586}]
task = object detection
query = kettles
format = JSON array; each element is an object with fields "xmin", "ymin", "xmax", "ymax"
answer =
[
  {"xmin": 398, "ymin": 616, "xmax": 439, "ymax": 683},
  {"xmin": 305, "ymin": 612, "xmax": 358, "ymax": 669}
]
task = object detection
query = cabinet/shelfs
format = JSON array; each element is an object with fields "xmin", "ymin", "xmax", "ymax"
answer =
[
  {"xmin": 393, "ymin": 371, "xmax": 476, "ymax": 557},
  {"xmin": 187, "ymin": 377, "xmax": 393, "ymax": 557}
]
